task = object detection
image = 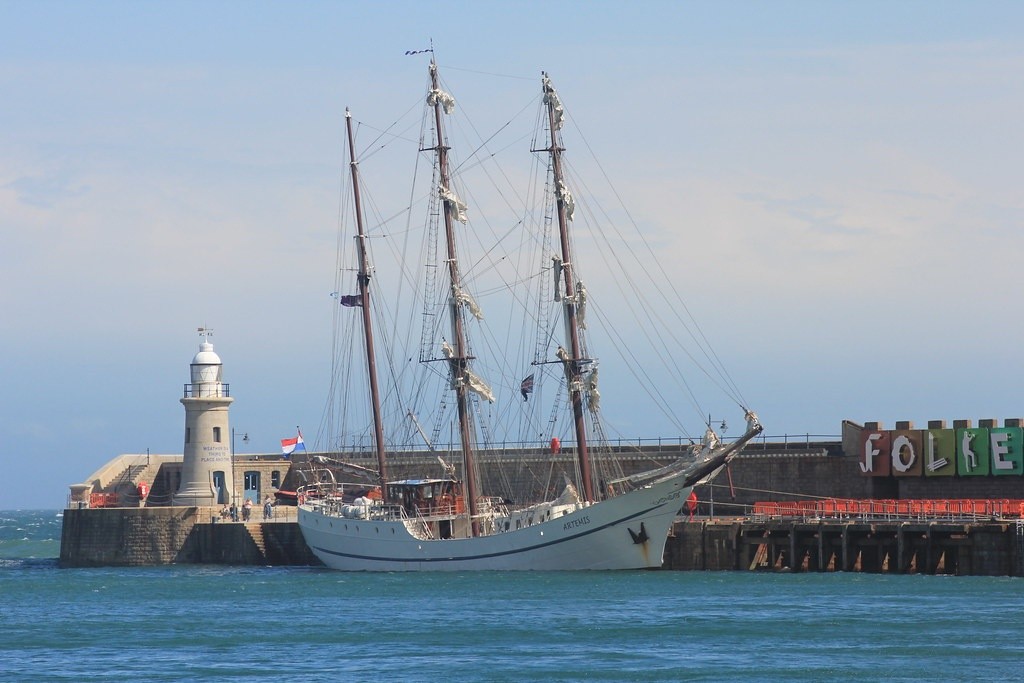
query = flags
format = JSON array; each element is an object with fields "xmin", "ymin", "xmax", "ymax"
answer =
[
  {"xmin": 342, "ymin": 293, "xmax": 370, "ymax": 308},
  {"xmin": 281, "ymin": 431, "xmax": 306, "ymax": 459},
  {"xmin": 521, "ymin": 374, "xmax": 533, "ymax": 402}
]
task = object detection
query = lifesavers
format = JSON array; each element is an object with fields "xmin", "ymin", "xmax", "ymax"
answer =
[
  {"xmin": 688, "ymin": 492, "xmax": 698, "ymax": 507},
  {"xmin": 138, "ymin": 481, "xmax": 149, "ymax": 499},
  {"xmin": 550, "ymin": 437, "xmax": 561, "ymax": 453}
]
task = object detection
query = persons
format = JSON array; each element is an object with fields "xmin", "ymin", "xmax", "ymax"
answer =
[
  {"xmin": 220, "ymin": 504, "xmax": 230, "ymax": 522},
  {"xmin": 230, "ymin": 503, "xmax": 240, "ymax": 521},
  {"xmin": 263, "ymin": 494, "xmax": 271, "ymax": 519},
  {"xmin": 242, "ymin": 496, "xmax": 252, "ymax": 521}
]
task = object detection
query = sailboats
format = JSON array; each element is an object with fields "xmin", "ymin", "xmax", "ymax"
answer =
[{"xmin": 296, "ymin": 38, "xmax": 762, "ymax": 571}]
